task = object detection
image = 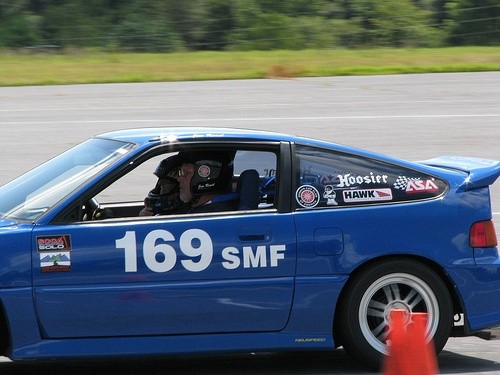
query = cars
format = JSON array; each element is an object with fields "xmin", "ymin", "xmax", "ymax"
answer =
[{"xmin": 0, "ymin": 125, "xmax": 500, "ymax": 375}]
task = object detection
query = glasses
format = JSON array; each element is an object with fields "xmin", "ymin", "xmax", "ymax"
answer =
[{"xmin": 178, "ymin": 170, "xmax": 195, "ymax": 177}]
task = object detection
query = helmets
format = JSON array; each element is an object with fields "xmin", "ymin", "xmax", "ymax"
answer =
[
  {"xmin": 144, "ymin": 155, "xmax": 181, "ymax": 213},
  {"xmin": 173, "ymin": 151, "xmax": 235, "ymax": 202}
]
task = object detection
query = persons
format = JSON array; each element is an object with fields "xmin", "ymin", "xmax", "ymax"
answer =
[
  {"xmin": 177, "ymin": 149, "xmax": 251, "ymax": 213},
  {"xmin": 138, "ymin": 154, "xmax": 184, "ymax": 216}
]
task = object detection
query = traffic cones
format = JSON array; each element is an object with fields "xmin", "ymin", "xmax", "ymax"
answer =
[{"xmin": 381, "ymin": 309, "xmax": 438, "ymax": 373}]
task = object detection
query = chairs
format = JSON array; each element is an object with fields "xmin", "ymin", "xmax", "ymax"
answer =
[{"xmin": 235, "ymin": 169, "xmax": 259, "ymax": 210}]
